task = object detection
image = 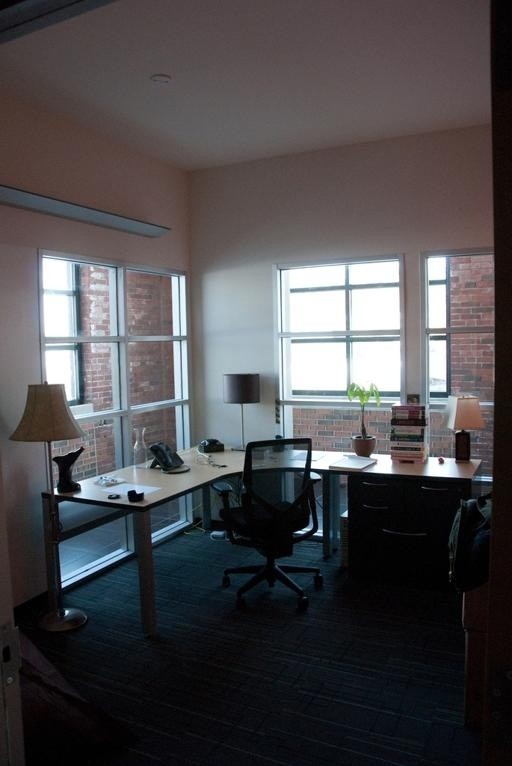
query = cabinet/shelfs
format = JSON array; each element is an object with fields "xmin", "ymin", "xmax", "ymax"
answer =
[{"xmin": 340, "ymin": 465, "xmax": 482, "ymax": 603}]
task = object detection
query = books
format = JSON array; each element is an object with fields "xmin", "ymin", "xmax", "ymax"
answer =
[
  {"xmin": 328, "ymin": 455, "xmax": 378, "ymax": 471},
  {"xmin": 390, "ymin": 403, "xmax": 430, "ymax": 464}
]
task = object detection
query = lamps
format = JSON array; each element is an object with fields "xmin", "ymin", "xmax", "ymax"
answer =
[
  {"xmin": 447, "ymin": 393, "xmax": 487, "ymax": 464},
  {"xmin": 8, "ymin": 383, "xmax": 89, "ymax": 636},
  {"xmin": 221, "ymin": 372, "xmax": 262, "ymax": 452}
]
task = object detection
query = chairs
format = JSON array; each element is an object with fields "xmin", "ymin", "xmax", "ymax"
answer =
[{"xmin": 221, "ymin": 438, "xmax": 326, "ymax": 610}]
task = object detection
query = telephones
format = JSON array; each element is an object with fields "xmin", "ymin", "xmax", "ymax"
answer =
[{"xmin": 149, "ymin": 442, "xmax": 184, "ymax": 471}]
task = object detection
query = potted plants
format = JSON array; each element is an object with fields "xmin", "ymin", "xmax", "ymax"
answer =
[{"xmin": 347, "ymin": 382, "xmax": 383, "ymax": 458}]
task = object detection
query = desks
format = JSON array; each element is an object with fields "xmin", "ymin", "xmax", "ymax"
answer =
[{"xmin": 38, "ymin": 441, "xmax": 485, "ymax": 638}]
